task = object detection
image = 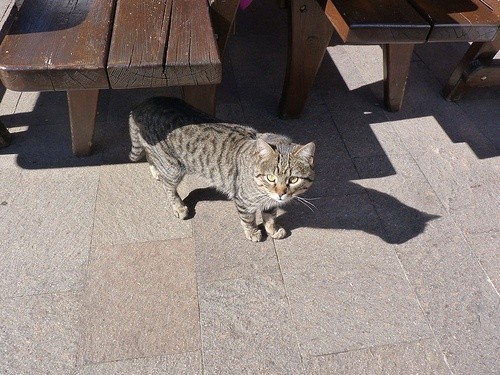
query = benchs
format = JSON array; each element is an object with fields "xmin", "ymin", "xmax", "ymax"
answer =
[
  {"xmin": 280, "ymin": 0, "xmax": 500, "ymax": 118},
  {"xmin": 0, "ymin": 0, "xmax": 241, "ymax": 156}
]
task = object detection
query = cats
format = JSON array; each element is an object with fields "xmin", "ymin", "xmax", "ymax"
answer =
[{"xmin": 128, "ymin": 96, "xmax": 319, "ymax": 243}]
